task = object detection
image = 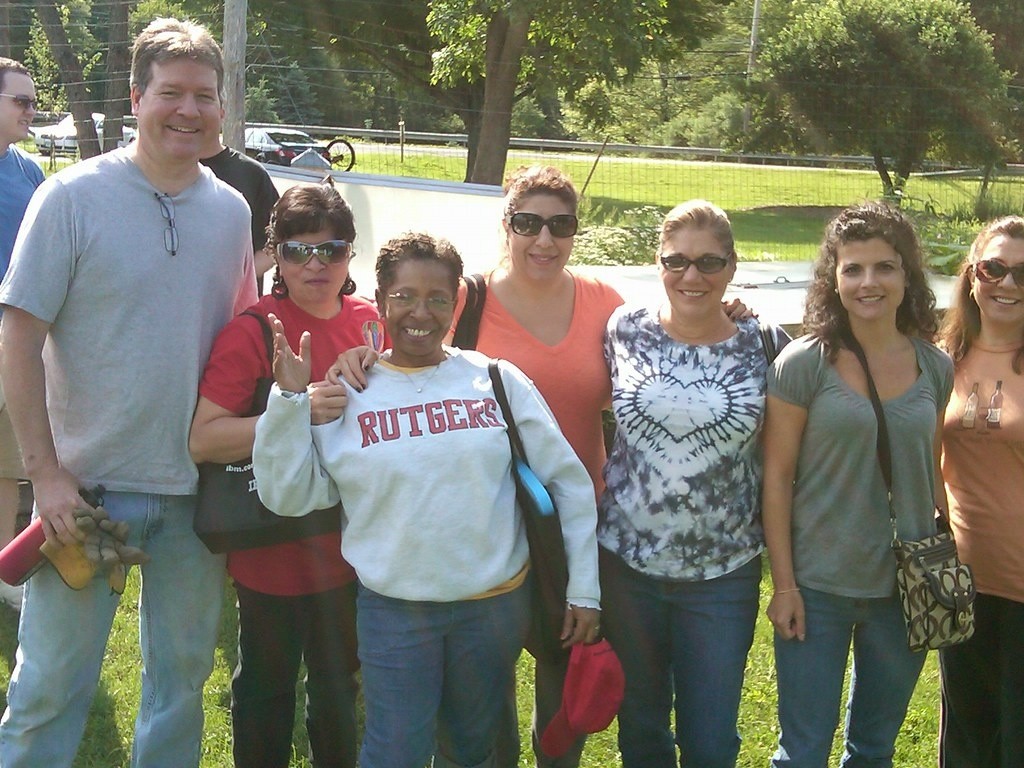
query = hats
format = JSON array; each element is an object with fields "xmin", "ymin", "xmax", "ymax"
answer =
[{"xmin": 540, "ymin": 635, "xmax": 624, "ymax": 760}]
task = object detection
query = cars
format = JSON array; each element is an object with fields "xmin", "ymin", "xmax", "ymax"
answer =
[
  {"xmin": 35, "ymin": 112, "xmax": 137, "ymax": 160},
  {"xmin": 245, "ymin": 126, "xmax": 331, "ymax": 169}
]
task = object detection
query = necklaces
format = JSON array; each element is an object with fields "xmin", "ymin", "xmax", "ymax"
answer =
[{"xmin": 386, "ymin": 356, "xmax": 446, "ymax": 394}]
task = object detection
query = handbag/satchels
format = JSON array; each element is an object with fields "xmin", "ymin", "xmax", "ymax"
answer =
[
  {"xmin": 192, "ymin": 312, "xmax": 342, "ymax": 555},
  {"xmin": 489, "ymin": 356, "xmax": 570, "ymax": 658},
  {"xmin": 891, "ymin": 515, "xmax": 975, "ymax": 651}
]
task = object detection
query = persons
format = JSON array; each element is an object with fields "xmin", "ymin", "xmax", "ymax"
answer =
[
  {"xmin": 930, "ymin": 214, "xmax": 1024, "ymax": 767},
  {"xmin": 252, "ymin": 229, "xmax": 603, "ymax": 768},
  {"xmin": 324, "ymin": 162, "xmax": 758, "ymax": 768},
  {"xmin": 0, "ymin": 16, "xmax": 279, "ymax": 768},
  {"xmin": 763, "ymin": 202, "xmax": 955, "ymax": 767},
  {"xmin": 188, "ymin": 182, "xmax": 395, "ymax": 768},
  {"xmin": 597, "ymin": 198, "xmax": 794, "ymax": 768}
]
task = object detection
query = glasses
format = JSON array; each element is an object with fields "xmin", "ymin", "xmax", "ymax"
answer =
[
  {"xmin": 0, "ymin": 94, "xmax": 37, "ymax": 109},
  {"xmin": 507, "ymin": 213, "xmax": 578, "ymax": 238},
  {"xmin": 660, "ymin": 253, "xmax": 732, "ymax": 274},
  {"xmin": 385, "ymin": 291, "xmax": 457, "ymax": 311},
  {"xmin": 276, "ymin": 239, "xmax": 350, "ymax": 265},
  {"xmin": 973, "ymin": 260, "xmax": 1024, "ymax": 286},
  {"xmin": 155, "ymin": 189, "xmax": 180, "ymax": 260}
]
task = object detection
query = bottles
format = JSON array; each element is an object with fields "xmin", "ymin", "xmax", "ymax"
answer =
[{"xmin": 0, "ymin": 483, "xmax": 106, "ymax": 587}]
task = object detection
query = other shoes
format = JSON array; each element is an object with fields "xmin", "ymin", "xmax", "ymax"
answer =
[{"xmin": 0, "ymin": 580, "xmax": 24, "ymax": 608}]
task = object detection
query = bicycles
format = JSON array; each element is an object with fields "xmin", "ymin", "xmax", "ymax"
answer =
[{"xmin": 324, "ymin": 140, "xmax": 356, "ymax": 173}]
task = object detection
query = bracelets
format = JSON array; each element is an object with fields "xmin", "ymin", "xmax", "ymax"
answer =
[{"xmin": 773, "ymin": 588, "xmax": 800, "ymax": 593}]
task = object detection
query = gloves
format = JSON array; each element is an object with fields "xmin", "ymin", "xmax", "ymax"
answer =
[{"xmin": 39, "ymin": 507, "xmax": 149, "ymax": 596}]
